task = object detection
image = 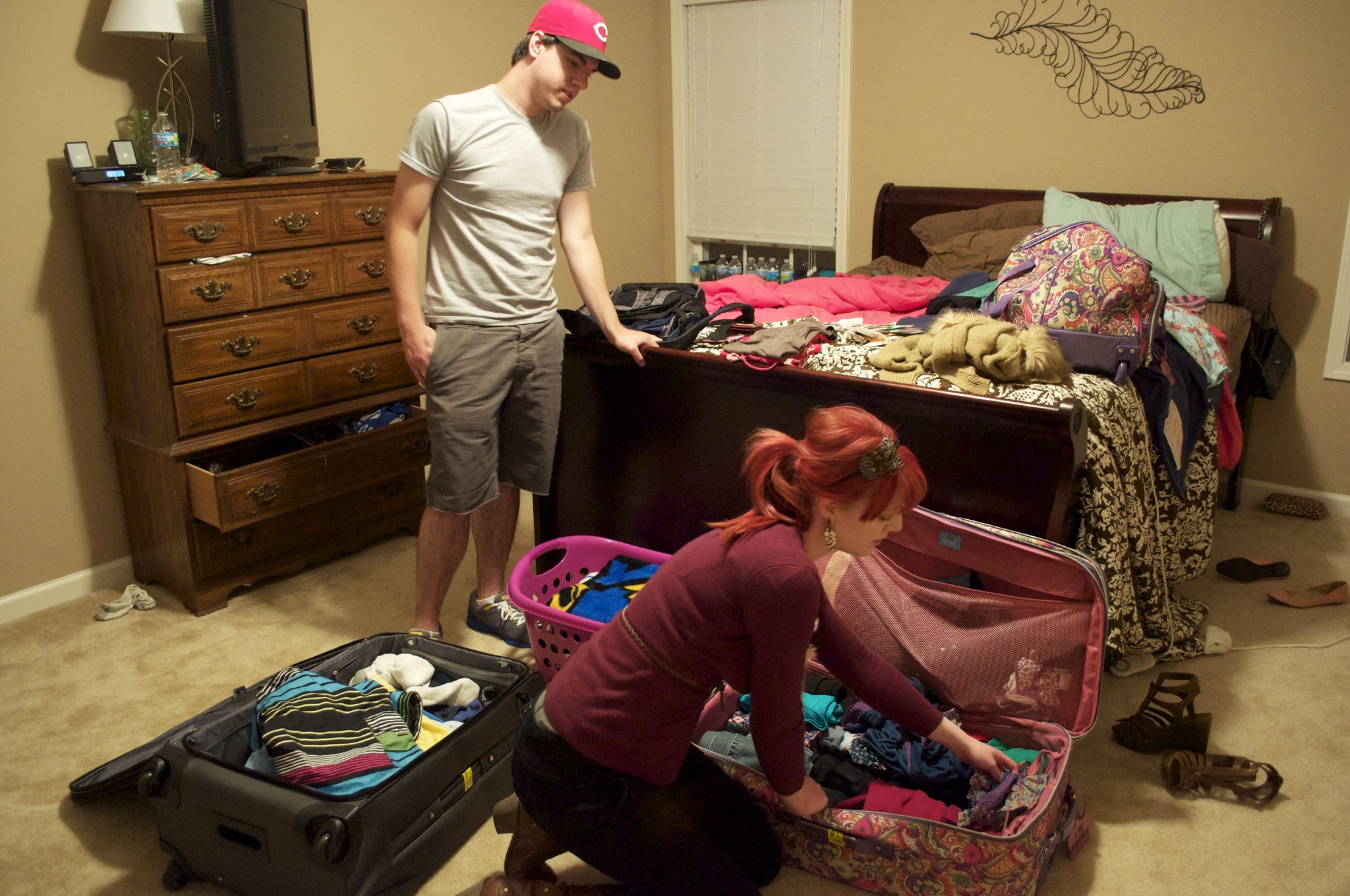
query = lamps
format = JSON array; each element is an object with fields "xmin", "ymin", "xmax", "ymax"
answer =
[
  {"xmin": 101, "ymin": 0, "xmax": 209, "ymax": 168},
  {"xmin": 63, "ymin": 139, "xmax": 149, "ymax": 184}
]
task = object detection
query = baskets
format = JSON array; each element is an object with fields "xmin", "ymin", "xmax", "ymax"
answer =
[{"xmin": 506, "ymin": 536, "xmax": 672, "ymax": 688}]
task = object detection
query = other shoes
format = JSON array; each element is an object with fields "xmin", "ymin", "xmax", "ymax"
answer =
[
  {"xmin": 1265, "ymin": 581, "xmax": 1348, "ymax": 608},
  {"xmin": 1214, "ymin": 558, "xmax": 1290, "ymax": 583}
]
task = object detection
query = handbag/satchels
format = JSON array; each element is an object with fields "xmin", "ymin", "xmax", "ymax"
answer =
[
  {"xmin": 982, "ymin": 223, "xmax": 1165, "ymax": 387},
  {"xmin": 721, "ymin": 333, "xmax": 834, "ymax": 371},
  {"xmin": 1242, "ymin": 305, "xmax": 1292, "ymax": 401},
  {"xmin": 723, "ymin": 319, "xmax": 833, "ymax": 361},
  {"xmin": 609, "ymin": 282, "xmax": 709, "ymax": 351}
]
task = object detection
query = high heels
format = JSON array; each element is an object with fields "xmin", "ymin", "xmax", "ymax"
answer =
[
  {"xmin": 1160, "ymin": 752, "xmax": 1283, "ymax": 806},
  {"xmin": 1112, "ymin": 672, "xmax": 1212, "ymax": 753}
]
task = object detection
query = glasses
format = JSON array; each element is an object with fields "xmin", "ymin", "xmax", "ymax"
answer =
[{"xmin": 320, "ymin": 158, "xmax": 366, "ymax": 174}]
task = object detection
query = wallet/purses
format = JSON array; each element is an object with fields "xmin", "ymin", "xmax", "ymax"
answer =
[
  {"xmin": 1265, "ymin": 493, "xmax": 1325, "ymax": 520},
  {"xmin": 729, "ymin": 322, "xmax": 764, "ymax": 331}
]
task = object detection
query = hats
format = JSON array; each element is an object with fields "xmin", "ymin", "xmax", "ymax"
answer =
[{"xmin": 526, "ymin": 0, "xmax": 622, "ymax": 81}]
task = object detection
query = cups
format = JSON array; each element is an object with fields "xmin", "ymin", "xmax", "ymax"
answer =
[{"xmin": 131, "ymin": 108, "xmax": 158, "ymax": 167}]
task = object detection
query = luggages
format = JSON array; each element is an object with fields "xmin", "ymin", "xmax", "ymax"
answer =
[
  {"xmin": 690, "ymin": 504, "xmax": 1109, "ymax": 896},
  {"xmin": 70, "ymin": 632, "xmax": 546, "ymax": 896}
]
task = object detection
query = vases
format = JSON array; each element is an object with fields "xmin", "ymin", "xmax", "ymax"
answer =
[{"xmin": 129, "ymin": 105, "xmax": 164, "ymax": 177}]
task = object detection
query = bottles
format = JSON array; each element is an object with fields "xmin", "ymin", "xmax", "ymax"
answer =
[
  {"xmin": 728, "ymin": 255, "xmax": 742, "ymax": 276},
  {"xmin": 755, "ymin": 257, "xmax": 766, "ymax": 281},
  {"xmin": 744, "ymin": 257, "xmax": 755, "ymax": 274},
  {"xmin": 780, "ymin": 259, "xmax": 794, "ymax": 284},
  {"xmin": 766, "ymin": 258, "xmax": 779, "ymax": 283},
  {"xmin": 151, "ymin": 111, "xmax": 182, "ymax": 186},
  {"xmin": 689, "ymin": 253, "xmax": 700, "ymax": 283},
  {"xmin": 716, "ymin": 254, "xmax": 728, "ymax": 281}
]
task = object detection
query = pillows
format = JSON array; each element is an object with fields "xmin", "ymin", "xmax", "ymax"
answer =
[
  {"xmin": 910, "ymin": 197, "xmax": 1046, "ymax": 261},
  {"xmin": 1228, "ymin": 230, "xmax": 1285, "ymax": 322},
  {"xmin": 1042, "ymin": 183, "xmax": 1231, "ymax": 302}
]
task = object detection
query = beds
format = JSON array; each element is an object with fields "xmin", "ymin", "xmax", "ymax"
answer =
[{"xmin": 532, "ymin": 182, "xmax": 1282, "ymax": 607}]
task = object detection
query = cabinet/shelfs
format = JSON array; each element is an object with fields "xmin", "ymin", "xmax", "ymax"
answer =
[{"xmin": 68, "ymin": 168, "xmax": 436, "ymax": 618}]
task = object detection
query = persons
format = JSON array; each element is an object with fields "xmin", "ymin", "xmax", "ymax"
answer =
[
  {"xmin": 387, "ymin": 0, "xmax": 663, "ymax": 654},
  {"xmin": 480, "ymin": 405, "xmax": 1019, "ymax": 896}
]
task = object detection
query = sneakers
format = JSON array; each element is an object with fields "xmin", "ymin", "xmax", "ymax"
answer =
[
  {"xmin": 466, "ymin": 589, "xmax": 531, "ymax": 644},
  {"xmin": 409, "ymin": 621, "xmax": 443, "ymax": 642}
]
